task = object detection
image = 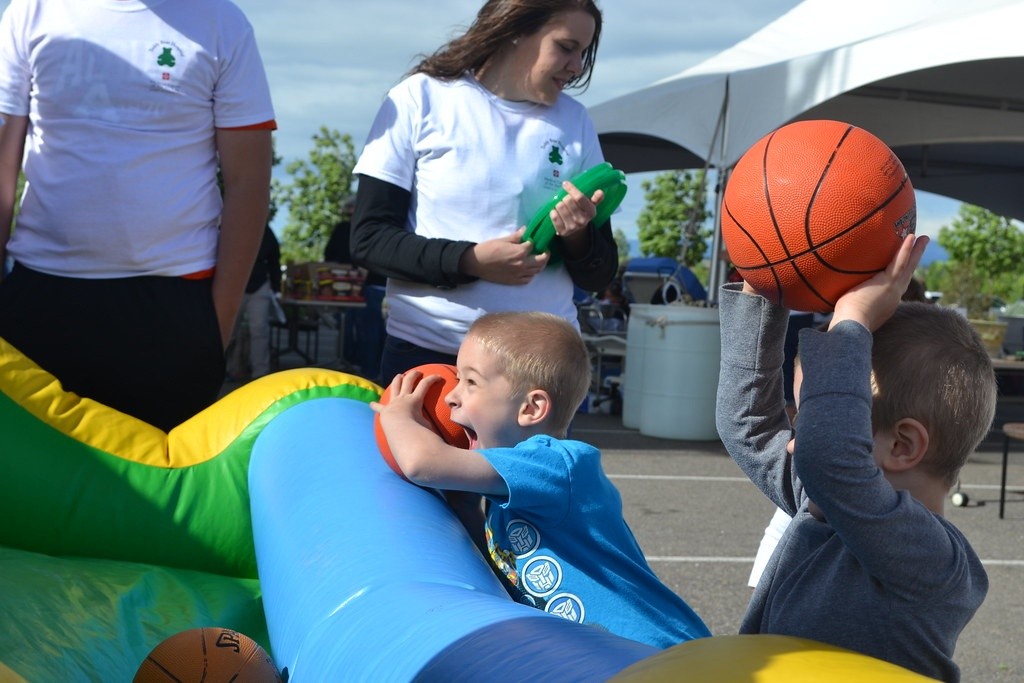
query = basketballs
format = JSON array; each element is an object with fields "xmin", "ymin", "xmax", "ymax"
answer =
[
  {"xmin": 133, "ymin": 628, "xmax": 282, "ymax": 683},
  {"xmin": 720, "ymin": 120, "xmax": 916, "ymax": 316},
  {"xmin": 374, "ymin": 364, "xmax": 477, "ymax": 486}
]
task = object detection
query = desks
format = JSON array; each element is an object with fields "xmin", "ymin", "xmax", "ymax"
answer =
[{"xmin": 276, "ymin": 293, "xmax": 368, "ymax": 374}]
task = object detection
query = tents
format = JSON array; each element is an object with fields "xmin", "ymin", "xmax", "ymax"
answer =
[{"xmin": 583, "ymin": 0, "xmax": 1024, "ymax": 306}]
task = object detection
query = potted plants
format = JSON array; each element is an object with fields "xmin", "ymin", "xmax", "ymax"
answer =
[{"xmin": 936, "ymin": 257, "xmax": 1010, "ymax": 359}]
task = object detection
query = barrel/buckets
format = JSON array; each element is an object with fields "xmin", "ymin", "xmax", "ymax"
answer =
[
  {"xmin": 619, "ymin": 301, "xmax": 651, "ymax": 430},
  {"xmin": 969, "ymin": 319, "xmax": 1009, "ymax": 359},
  {"xmin": 639, "ymin": 304, "xmax": 723, "ymax": 441},
  {"xmin": 997, "ymin": 315, "xmax": 1024, "ymax": 355}
]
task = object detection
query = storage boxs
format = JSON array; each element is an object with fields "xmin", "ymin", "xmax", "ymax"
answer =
[{"xmin": 285, "ymin": 262, "xmax": 368, "ymax": 303}]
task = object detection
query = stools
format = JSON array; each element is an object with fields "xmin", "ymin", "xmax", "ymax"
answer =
[{"xmin": 269, "ymin": 320, "xmax": 319, "ymax": 368}]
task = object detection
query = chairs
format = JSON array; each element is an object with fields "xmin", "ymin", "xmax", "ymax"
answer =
[{"xmin": 998, "ymin": 423, "xmax": 1024, "ymax": 520}]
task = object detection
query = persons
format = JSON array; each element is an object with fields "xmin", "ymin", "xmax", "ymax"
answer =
[
  {"xmin": 280, "ymin": 0, "xmax": 716, "ymax": 651},
  {"xmin": 0, "ymin": 0, "xmax": 277, "ymax": 434},
  {"xmin": 715, "ymin": 277, "xmax": 997, "ymax": 683}
]
